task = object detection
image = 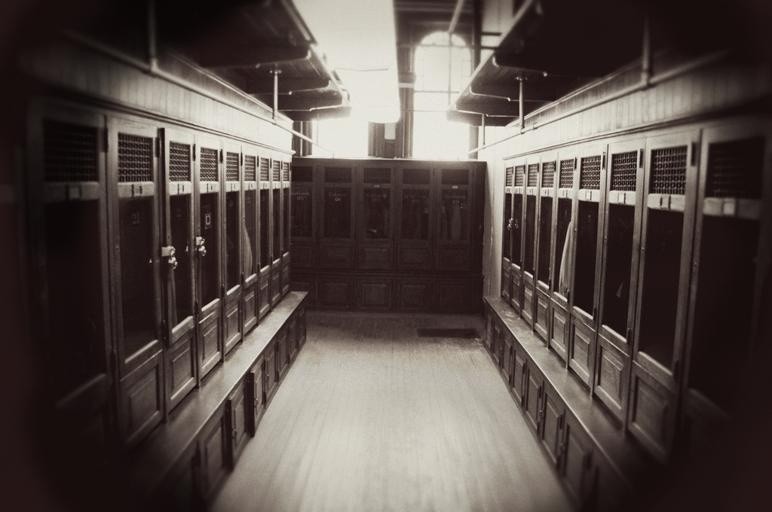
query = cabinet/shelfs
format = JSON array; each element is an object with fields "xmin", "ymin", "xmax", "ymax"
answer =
[
  {"xmin": 287, "ymin": 158, "xmax": 318, "ymax": 268},
  {"xmin": 136, "ymin": 290, "xmax": 312, "ymax": 512},
  {"xmin": 396, "ymin": 162, "xmax": 484, "ymax": 273},
  {"xmin": 317, "ymin": 159, "xmax": 397, "ymax": 270},
  {"xmin": 0, "ymin": 86, "xmax": 120, "ymax": 491},
  {"xmin": 195, "ymin": 126, "xmax": 242, "ymax": 384},
  {"xmin": 289, "ymin": 269, "xmax": 481, "ymax": 314},
  {"xmin": 478, "ymin": 298, "xmax": 657, "ymax": 511},
  {"xmin": 238, "ymin": 141, "xmax": 271, "ymax": 339},
  {"xmin": 271, "ymin": 144, "xmax": 291, "ymax": 307},
  {"xmin": 104, "ymin": 105, "xmax": 198, "ymax": 456}
]
